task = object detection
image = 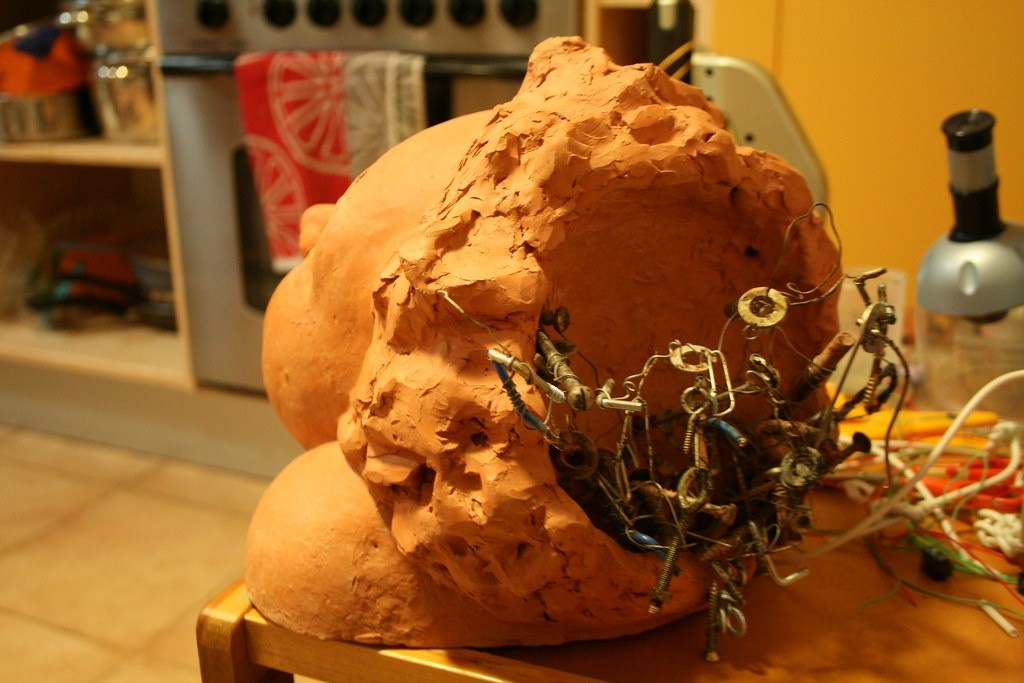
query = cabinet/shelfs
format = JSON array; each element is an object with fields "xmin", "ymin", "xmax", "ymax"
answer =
[{"xmin": 2, "ymin": 2, "xmax": 193, "ymax": 398}]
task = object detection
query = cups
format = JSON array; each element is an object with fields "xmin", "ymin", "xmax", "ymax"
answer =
[{"xmin": 835, "ymin": 265, "xmax": 906, "ymax": 396}]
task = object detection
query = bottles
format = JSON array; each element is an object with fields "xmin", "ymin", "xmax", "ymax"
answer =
[{"xmin": 913, "ymin": 107, "xmax": 1022, "ymax": 419}]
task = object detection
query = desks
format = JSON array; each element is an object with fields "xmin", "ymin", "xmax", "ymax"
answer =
[{"xmin": 194, "ymin": 420, "xmax": 1022, "ymax": 681}]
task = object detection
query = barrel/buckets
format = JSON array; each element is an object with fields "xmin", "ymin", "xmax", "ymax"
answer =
[
  {"xmin": 1, "ymin": 4, "xmax": 92, "ymax": 144},
  {"xmin": 87, "ymin": 41, "xmax": 160, "ymax": 142}
]
